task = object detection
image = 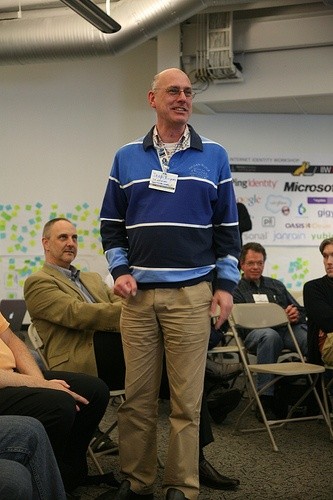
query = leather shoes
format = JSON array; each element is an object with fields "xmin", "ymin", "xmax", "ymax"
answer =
[
  {"xmin": 204, "ymin": 359, "xmax": 244, "ymax": 383},
  {"xmin": 199, "ymin": 460, "xmax": 241, "ymax": 490}
]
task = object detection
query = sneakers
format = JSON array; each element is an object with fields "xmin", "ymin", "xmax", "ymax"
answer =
[{"xmin": 88, "ymin": 431, "xmax": 118, "ymax": 455}]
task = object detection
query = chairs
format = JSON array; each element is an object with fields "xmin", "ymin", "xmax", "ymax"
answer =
[
  {"xmin": 25, "ymin": 323, "xmax": 165, "ymax": 477},
  {"xmin": 208, "ymin": 301, "xmax": 333, "ymax": 451}
]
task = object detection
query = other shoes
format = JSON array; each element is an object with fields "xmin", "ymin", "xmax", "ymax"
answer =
[
  {"xmin": 166, "ymin": 488, "xmax": 190, "ymax": 500},
  {"xmin": 94, "ymin": 480, "xmax": 155, "ymax": 500},
  {"xmin": 209, "ymin": 393, "xmax": 242, "ymax": 425},
  {"xmin": 256, "ymin": 399, "xmax": 276, "ymax": 423},
  {"xmin": 205, "ymin": 387, "xmax": 241, "ymax": 404}
]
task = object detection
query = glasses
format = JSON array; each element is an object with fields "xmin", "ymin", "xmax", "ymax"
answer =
[{"xmin": 167, "ymin": 89, "xmax": 197, "ymax": 100}]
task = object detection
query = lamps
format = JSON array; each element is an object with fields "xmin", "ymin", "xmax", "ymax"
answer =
[{"xmin": 60, "ymin": 0, "xmax": 121, "ymax": 34}]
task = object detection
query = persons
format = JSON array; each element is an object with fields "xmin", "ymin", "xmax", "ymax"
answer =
[
  {"xmin": 303, "ymin": 237, "xmax": 333, "ymax": 373},
  {"xmin": 101, "ymin": 68, "xmax": 241, "ymax": 500},
  {"xmin": 23, "ymin": 218, "xmax": 241, "ymax": 489},
  {"xmin": 0, "ymin": 310, "xmax": 110, "ymax": 500},
  {"xmin": 234, "ymin": 242, "xmax": 306, "ymax": 421}
]
